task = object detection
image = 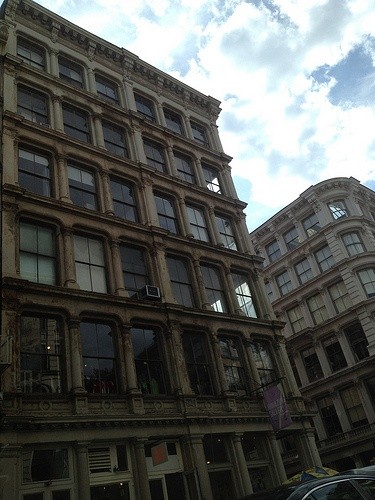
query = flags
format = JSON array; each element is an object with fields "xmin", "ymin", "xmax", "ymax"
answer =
[{"xmin": 261, "ymin": 381, "xmax": 293, "ymax": 435}]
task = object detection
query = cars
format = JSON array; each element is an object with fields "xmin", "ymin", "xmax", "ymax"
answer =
[
  {"xmin": 334, "ymin": 465, "xmax": 375, "ymax": 476},
  {"xmin": 235, "ymin": 474, "xmax": 375, "ymax": 500}
]
